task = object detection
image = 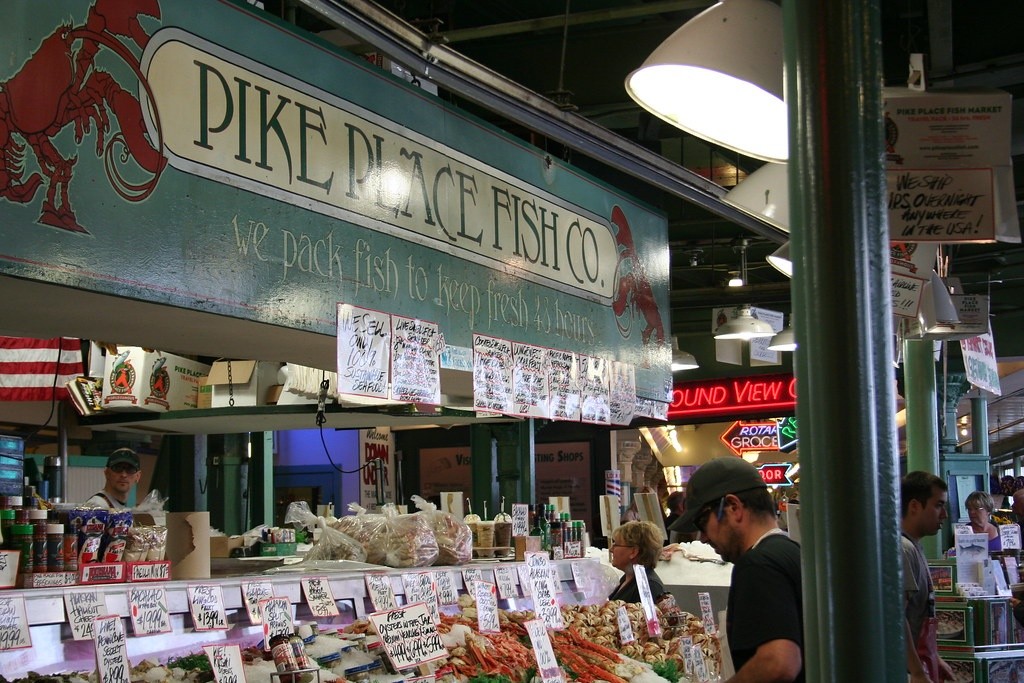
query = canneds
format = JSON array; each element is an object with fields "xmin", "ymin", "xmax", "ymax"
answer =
[
  {"xmin": 549, "ymin": 521, "xmax": 563, "ymax": 559},
  {"xmin": 0, "ymin": 495, "xmax": 77, "ymax": 573},
  {"xmin": 655, "ymin": 591, "xmax": 684, "ymax": 626},
  {"xmin": 269, "ymin": 633, "xmax": 315, "ymax": 683}
]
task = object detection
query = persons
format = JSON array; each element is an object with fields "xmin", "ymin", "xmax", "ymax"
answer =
[
  {"xmin": 620, "ymin": 485, "xmax": 655, "ymax": 522},
  {"xmin": 608, "ymin": 520, "xmax": 665, "ymax": 603},
  {"xmin": 1011, "ymin": 489, "xmax": 1024, "ymax": 549},
  {"xmin": 667, "ymin": 456, "xmax": 806, "ymax": 683},
  {"xmin": 86, "ymin": 448, "xmax": 141, "ymax": 508},
  {"xmin": 901, "ymin": 471, "xmax": 957, "ymax": 683},
  {"xmin": 950, "ymin": 491, "xmax": 1001, "ymax": 549},
  {"xmin": 665, "ymin": 491, "xmax": 691, "ymax": 543},
  {"xmin": 1010, "ymin": 597, "xmax": 1024, "ymax": 627}
]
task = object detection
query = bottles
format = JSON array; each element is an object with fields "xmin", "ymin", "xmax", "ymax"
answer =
[
  {"xmin": 529, "ymin": 502, "xmax": 587, "ymax": 559},
  {"xmin": 270, "ymin": 632, "xmax": 314, "ymax": 683},
  {"xmin": 0, "ymin": 494, "xmax": 79, "ymax": 576}
]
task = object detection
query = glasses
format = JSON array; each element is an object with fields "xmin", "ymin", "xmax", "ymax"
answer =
[
  {"xmin": 611, "ymin": 541, "xmax": 639, "ymax": 548},
  {"xmin": 694, "ymin": 498, "xmax": 742, "ymax": 534},
  {"xmin": 964, "ymin": 506, "xmax": 985, "ymax": 513},
  {"xmin": 109, "ymin": 466, "xmax": 139, "ymax": 474}
]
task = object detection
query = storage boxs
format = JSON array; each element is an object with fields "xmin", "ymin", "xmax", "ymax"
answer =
[
  {"xmin": 264, "ymin": 542, "xmax": 298, "ymax": 556},
  {"xmin": 80, "ymin": 560, "xmax": 126, "ymax": 584},
  {"xmin": 209, "ymin": 536, "xmax": 244, "ymax": 557},
  {"xmin": 126, "ymin": 552, "xmax": 173, "ymax": 580},
  {"xmin": 100, "ymin": 347, "xmax": 285, "ymax": 412}
]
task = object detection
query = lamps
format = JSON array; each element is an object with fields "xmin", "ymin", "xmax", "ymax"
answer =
[
  {"xmin": 765, "ymin": 240, "xmax": 793, "ymax": 278},
  {"xmin": 728, "ymin": 271, "xmax": 743, "ymax": 286},
  {"xmin": 720, "ymin": 162, "xmax": 791, "ymax": 234},
  {"xmin": 669, "ymin": 266, "xmax": 700, "ymax": 370},
  {"xmin": 922, "ymin": 270, "xmax": 960, "ymax": 332},
  {"xmin": 624, "ymin": 0, "xmax": 789, "ymax": 167},
  {"xmin": 766, "ymin": 313, "xmax": 798, "ymax": 352},
  {"xmin": 714, "ymin": 239, "xmax": 778, "ymax": 339}
]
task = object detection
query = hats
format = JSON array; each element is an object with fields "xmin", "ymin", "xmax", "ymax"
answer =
[
  {"xmin": 669, "ymin": 456, "xmax": 767, "ymax": 534},
  {"xmin": 667, "ymin": 491, "xmax": 683, "ymax": 509},
  {"xmin": 106, "ymin": 448, "xmax": 140, "ymax": 471}
]
